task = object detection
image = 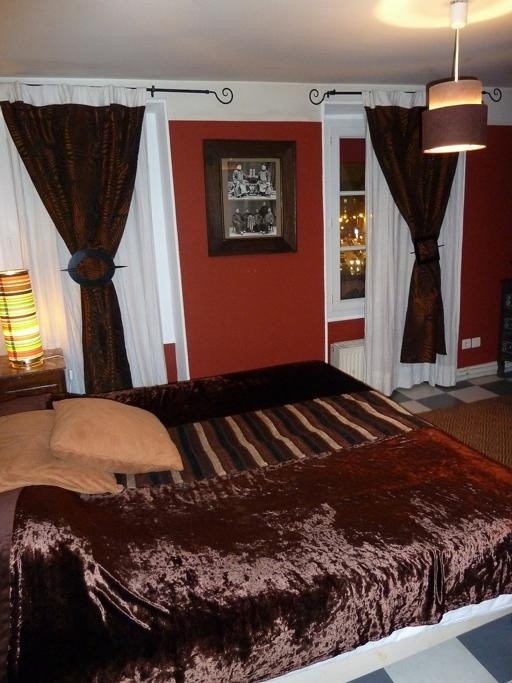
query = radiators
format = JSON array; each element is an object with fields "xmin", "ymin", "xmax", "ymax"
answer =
[{"xmin": 330, "ymin": 338, "xmax": 367, "ymax": 384}]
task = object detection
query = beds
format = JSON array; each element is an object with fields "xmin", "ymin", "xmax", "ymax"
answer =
[{"xmin": 0, "ymin": 360, "xmax": 512, "ymax": 683}]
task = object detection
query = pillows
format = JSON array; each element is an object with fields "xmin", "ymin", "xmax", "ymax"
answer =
[
  {"xmin": 48, "ymin": 398, "xmax": 183, "ymax": 472},
  {"xmin": 0, "ymin": 410, "xmax": 131, "ymax": 501}
]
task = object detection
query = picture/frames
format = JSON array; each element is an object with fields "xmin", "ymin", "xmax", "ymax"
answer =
[{"xmin": 203, "ymin": 138, "xmax": 297, "ymax": 257}]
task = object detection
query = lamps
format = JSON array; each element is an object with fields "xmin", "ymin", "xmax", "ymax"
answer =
[
  {"xmin": 422, "ymin": 1, "xmax": 488, "ymax": 156},
  {"xmin": 1, "ymin": 269, "xmax": 44, "ymax": 368}
]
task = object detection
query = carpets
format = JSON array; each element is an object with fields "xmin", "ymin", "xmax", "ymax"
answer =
[{"xmin": 414, "ymin": 394, "xmax": 512, "ymax": 470}]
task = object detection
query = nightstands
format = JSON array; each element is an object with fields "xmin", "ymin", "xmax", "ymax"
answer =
[{"xmin": 0, "ymin": 348, "xmax": 69, "ymax": 400}]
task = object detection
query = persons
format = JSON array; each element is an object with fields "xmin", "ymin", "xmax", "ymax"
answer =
[
  {"xmin": 232, "ymin": 201, "xmax": 274, "ymax": 235},
  {"xmin": 257, "ymin": 164, "xmax": 272, "ymax": 197},
  {"xmin": 230, "ymin": 165, "xmax": 249, "ymax": 198}
]
